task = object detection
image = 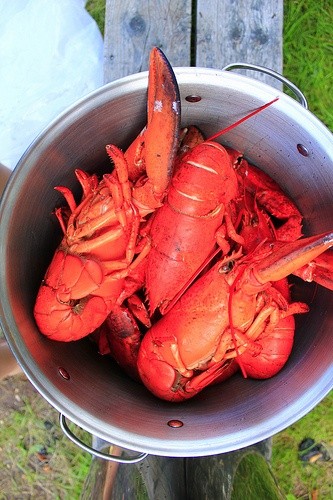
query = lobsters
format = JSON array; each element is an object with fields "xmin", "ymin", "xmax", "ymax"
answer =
[
  {"xmin": 235, "ymin": 272, "xmax": 308, "ymax": 378},
  {"xmin": 94, "ymin": 98, "xmax": 281, "ymax": 367},
  {"xmin": 137, "ymin": 228, "xmax": 333, "ymax": 401},
  {"xmin": 35, "ymin": 45, "xmax": 181, "ymax": 341}
]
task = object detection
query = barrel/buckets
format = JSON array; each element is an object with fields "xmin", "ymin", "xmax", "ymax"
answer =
[{"xmin": 1, "ymin": 57, "xmax": 333, "ymax": 463}]
